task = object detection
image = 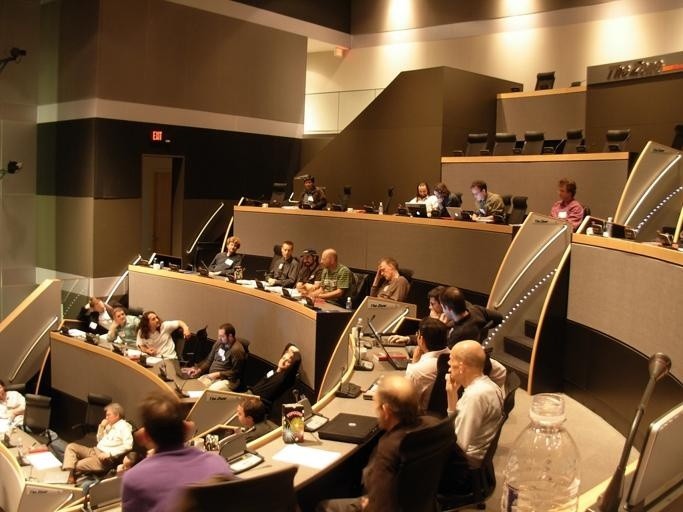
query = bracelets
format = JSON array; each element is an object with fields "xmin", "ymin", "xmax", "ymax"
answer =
[{"xmin": 478, "ymin": 216, "xmax": 480, "ymax": 220}]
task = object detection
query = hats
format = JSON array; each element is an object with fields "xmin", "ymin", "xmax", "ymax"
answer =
[{"xmin": 300, "ymin": 249, "xmax": 315, "ymax": 257}]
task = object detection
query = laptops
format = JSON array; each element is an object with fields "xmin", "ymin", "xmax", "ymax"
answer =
[
  {"xmin": 368, "ymin": 318, "xmax": 407, "ymax": 346},
  {"xmin": 172, "ymin": 360, "xmax": 198, "ymax": 380},
  {"xmin": 318, "ymin": 413, "xmax": 378, "ymax": 442},
  {"xmin": 381, "ymin": 345, "xmax": 412, "ymax": 370},
  {"xmin": 446, "ymin": 207, "xmax": 464, "ymax": 220},
  {"xmin": 407, "ymin": 204, "xmax": 431, "ymax": 218}
]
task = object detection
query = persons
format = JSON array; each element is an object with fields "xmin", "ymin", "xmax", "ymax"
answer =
[
  {"xmin": 60, "ymin": 402, "xmax": 134, "ymax": 484},
  {"xmin": 547, "ymin": 176, "xmax": 585, "ymax": 233},
  {"xmin": 120, "ymin": 389, "xmax": 233, "ymax": 511},
  {"xmin": 298, "ymin": 248, "xmax": 357, "ymax": 305},
  {"xmin": 76, "ymin": 285, "xmax": 506, "ymax": 459},
  {"xmin": 469, "ymin": 179, "xmax": 505, "ymax": 220},
  {"xmin": 0, "ymin": 379, "xmax": 25, "ymax": 431},
  {"xmin": 296, "ymin": 175, "xmax": 326, "ymax": 209},
  {"xmin": 368, "ymin": 256, "xmax": 410, "ymax": 303},
  {"xmin": 292, "ymin": 247, "xmax": 322, "ymax": 293},
  {"xmin": 407, "ymin": 182, "xmax": 438, "ymax": 213},
  {"xmin": 263, "ymin": 241, "xmax": 299, "ymax": 287},
  {"xmin": 116, "ymin": 451, "xmax": 146, "ymax": 474},
  {"xmin": 206, "ymin": 236, "xmax": 242, "ymax": 277},
  {"xmin": 317, "ymin": 371, "xmax": 491, "ymax": 510},
  {"xmin": 429, "ymin": 182, "xmax": 460, "ymax": 217}
]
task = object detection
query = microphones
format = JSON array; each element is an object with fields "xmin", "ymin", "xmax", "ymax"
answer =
[
  {"xmin": 354, "ymin": 327, "xmax": 374, "ymax": 370},
  {"xmin": 219, "ymin": 426, "xmax": 257, "ymax": 456},
  {"xmin": 304, "ymin": 285, "xmax": 327, "ymax": 310},
  {"xmin": 336, "ymin": 366, "xmax": 361, "ymax": 398},
  {"xmin": 293, "ymin": 389, "xmax": 299, "ymax": 404},
  {"xmin": 158, "ymin": 355, "xmax": 174, "ymax": 382},
  {"xmin": 18, "ymin": 442, "xmax": 37, "ymax": 466},
  {"xmin": 138, "ymin": 345, "xmax": 154, "ymax": 368},
  {"xmin": 200, "ymin": 260, "xmax": 213, "ymax": 278},
  {"xmin": 1, "ymin": 419, "xmax": 23, "ymax": 448},
  {"xmin": 362, "ymin": 314, "xmax": 376, "ymax": 334},
  {"xmin": 587, "ymin": 352, "xmax": 671, "ymax": 512},
  {"xmin": 81, "ymin": 466, "xmax": 113, "ymax": 512},
  {"xmin": 175, "ymin": 370, "xmax": 194, "ymax": 398}
]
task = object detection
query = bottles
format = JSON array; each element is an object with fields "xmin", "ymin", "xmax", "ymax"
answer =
[
  {"xmin": 194, "ymin": 437, "xmax": 205, "ymax": 450},
  {"xmin": 152, "ymin": 258, "xmax": 157, "ymax": 269},
  {"xmin": 605, "ymin": 216, "xmax": 613, "ymax": 234},
  {"xmin": 356, "ymin": 317, "xmax": 364, "ymax": 337},
  {"xmin": 495, "ymin": 392, "xmax": 580, "ymax": 512},
  {"xmin": 15, "ymin": 436, "xmax": 23, "ymax": 453},
  {"xmin": 378, "ymin": 202, "xmax": 384, "ymax": 216},
  {"xmin": 122, "ymin": 341, "xmax": 129, "ymax": 357},
  {"xmin": 345, "ymin": 296, "xmax": 352, "ymax": 311}
]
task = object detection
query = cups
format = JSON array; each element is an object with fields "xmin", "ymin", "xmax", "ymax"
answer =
[
  {"xmin": 346, "ymin": 207, "xmax": 354, "ymax": 213},
  {"xmin": 261, "ymin": 202, "xmax": 269, "ymax": 208}
]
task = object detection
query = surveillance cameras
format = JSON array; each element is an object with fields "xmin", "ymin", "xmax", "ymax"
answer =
[{"xmin": 10, "ymin": 46, "xmax": 27, "ymax": 57}]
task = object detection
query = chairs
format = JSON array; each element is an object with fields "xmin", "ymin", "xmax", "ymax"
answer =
[
  {"xmin": 449, "ymin": 129, "xmax": 630, "ymax": 156},
  {"xmin": 671, "ymin": 125, "xmax": 683, "ymax": 150},
  {"xmin": 535, "ymin": 72, "xmax": 555, "ymax": 90}
]
